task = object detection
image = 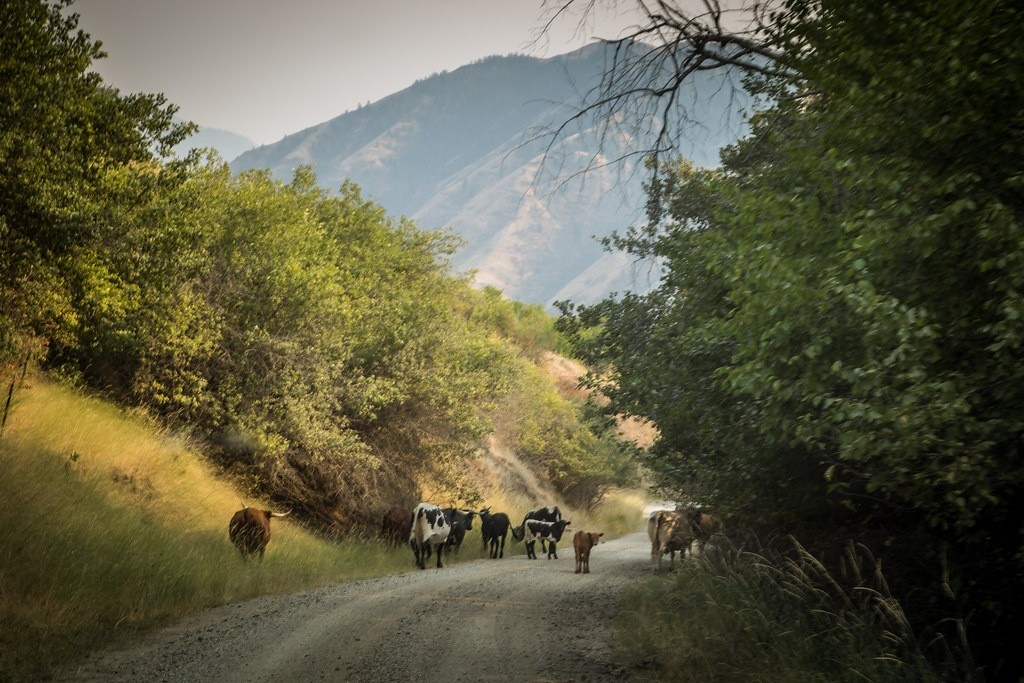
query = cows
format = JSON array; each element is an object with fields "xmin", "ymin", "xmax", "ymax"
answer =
[
  {"xmin": 228, "ymin": 498, "xmax": 295, "ymax": 567},
  {"xmin": 376, "ymin": 501, "xmax": 727, "ymax": 575}
]
